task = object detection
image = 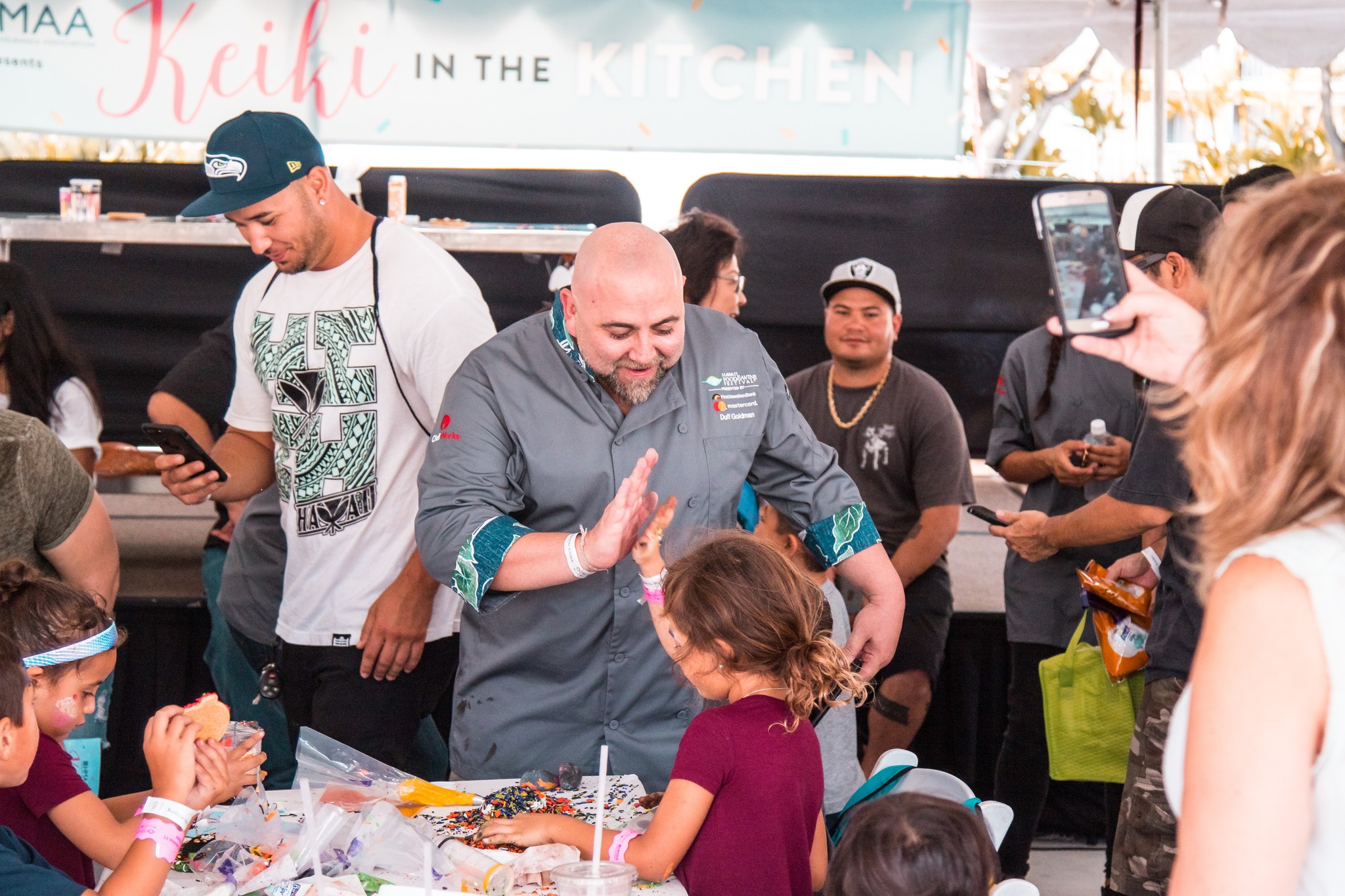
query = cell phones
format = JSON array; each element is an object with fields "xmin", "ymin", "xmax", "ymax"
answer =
[
  {"xmin": 140, "ymin": 421, "xmax": 229, "ymax": 483},
  {"xmin": 967, "ymin": 506, "xmax": 1011, "ymax": 527},
  {"xmin": 1031, "ymin": 185, "xmax": 1137, "ymax": 338}
]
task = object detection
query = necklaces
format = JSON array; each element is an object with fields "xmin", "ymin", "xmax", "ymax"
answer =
[{"xmin": 827, "ymin": 361, "xmax": 890, "ymax": 429}]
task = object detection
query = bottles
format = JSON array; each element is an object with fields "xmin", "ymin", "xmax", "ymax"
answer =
[{"xmin": 1083, "ymin": 418, "xmax": 1118, "ymax": 503}]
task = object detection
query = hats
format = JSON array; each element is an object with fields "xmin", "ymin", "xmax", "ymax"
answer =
[
  {"xmin": 181, "ymin": 110, "xmax": 325, "ymax": 216},
  {"xmin": 1117, "ymin": 183, "xmax": 1224, "ymax": 261},
  {"xmin": 821, "ymin": 253, "xmax": 901, "ymax": 315}
]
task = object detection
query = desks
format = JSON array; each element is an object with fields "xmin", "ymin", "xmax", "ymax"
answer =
[{"xmin": 0, "ymin": 206, "xmax": 595, "ymax": 264}]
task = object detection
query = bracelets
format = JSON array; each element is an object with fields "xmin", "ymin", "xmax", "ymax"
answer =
[
  {"xmin": 606, "ymin": 828, "xmax": 644, "ymax": 864},
  {"xmin": 563, "ymin": 524, "xmax": 609, "ymax": 579},
  {"xmin": 134, "ymin": 794, "xmax": 195, "ymax": 863},
  {"xmin": 635, "ymin": 567, "xmax": 672, "ymax": 606}
]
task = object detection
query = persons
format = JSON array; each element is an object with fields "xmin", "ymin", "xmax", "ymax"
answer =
[
  {"xmin": 414, "ymin": 221, "xmax": 905, "ymax": 798},
  {"xmin": 986, "ymin": 179, "xmax": 1225, "ymax": 896},
  {"xmin": 150, "ymin": 110, "xmax": 500, "ymax": 775},
  {"xmin": 817, "ymin": 789, "xmax": 1040, "ymax": 896},
  {"xmin": 1136, "ymin": 176, "xmax": 1345, "ymax": 896},
  {"xmin": 744, "ymin": 500, "xmax": 870, "ymax": 842},
  {"xmin": 0, "ymin": 258, "xmax": 103, "ymax": 478},
  {"xmin": 479, "ymin": 495, "xmax": 875, "ymax": 896},
  {"xmin": 984, "ymin": 312, "xmax": 1152, "ymax": 882},
  {"xmin": 656, "ymin": 203, "xmax": 763, "ymax": 534},
  {"xmin": 1, "ymin": 407, "xmax": 122, "ymax": 759},
  {"xmin": 0, "ymin": 557, "xmax": 267, "ymax": 890},
  {"xmin": 785, "ymin": 256, "xmax": 976, "ymax": 784},
  {"xmin": 214, "ymin": 472, "xmax": 287, "ymax": 696},
  {"xmin": 0, "ymin": 630, "xmax": 231, "ymax": 896},
  {"xmin": 145, "ymin": 312, "xmax": 451, "ymax": 792}
]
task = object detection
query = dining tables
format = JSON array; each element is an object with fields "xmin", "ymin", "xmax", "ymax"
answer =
[{"xmin": 78, "ymin": 751, "xmax": 698, "ymax": 896}]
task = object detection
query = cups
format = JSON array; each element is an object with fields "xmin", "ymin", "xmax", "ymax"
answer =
[
  {"xmin": 59, "ymin": 177, "xmax": 103, "ymax": 222},
  {"xmin": 428, "ymin": 832, "xmax": 519, "ymax": 895},
  {"xmin": 549, "ymin": 861, "xmax": 639, "ymax": 896}
]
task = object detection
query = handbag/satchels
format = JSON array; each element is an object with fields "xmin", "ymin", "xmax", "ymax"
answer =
[{"xmin": 1038, "ymin": 604, "xmax": 1146, "ymax": 782}]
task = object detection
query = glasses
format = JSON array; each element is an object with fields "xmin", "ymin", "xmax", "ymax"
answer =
[
  {"xmin": 715, "ymin": 273, "xmax": 745, "ymax": 294},
  {"xmin": 1134, "ymin": 250, "xmax": 1167, "ymax": 274}
]
